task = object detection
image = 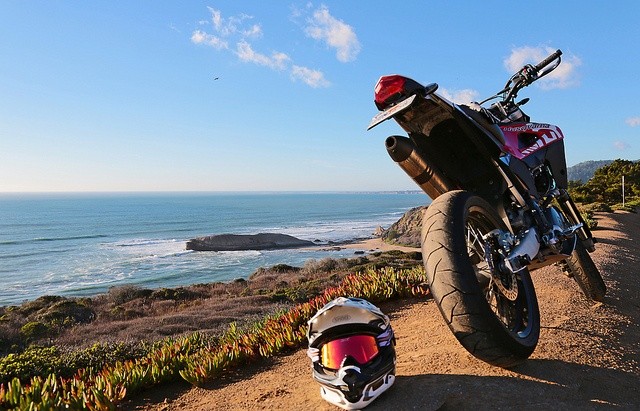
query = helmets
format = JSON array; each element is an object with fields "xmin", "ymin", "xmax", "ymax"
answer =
[{"xmin": 306, "ymin": 297, "xmax": 396, "ymax": 411}]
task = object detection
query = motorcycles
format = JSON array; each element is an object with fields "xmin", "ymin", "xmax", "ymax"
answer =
[{"xmin": 366, "ymin": 48, "xmax": 608, "ymax": 370}]
